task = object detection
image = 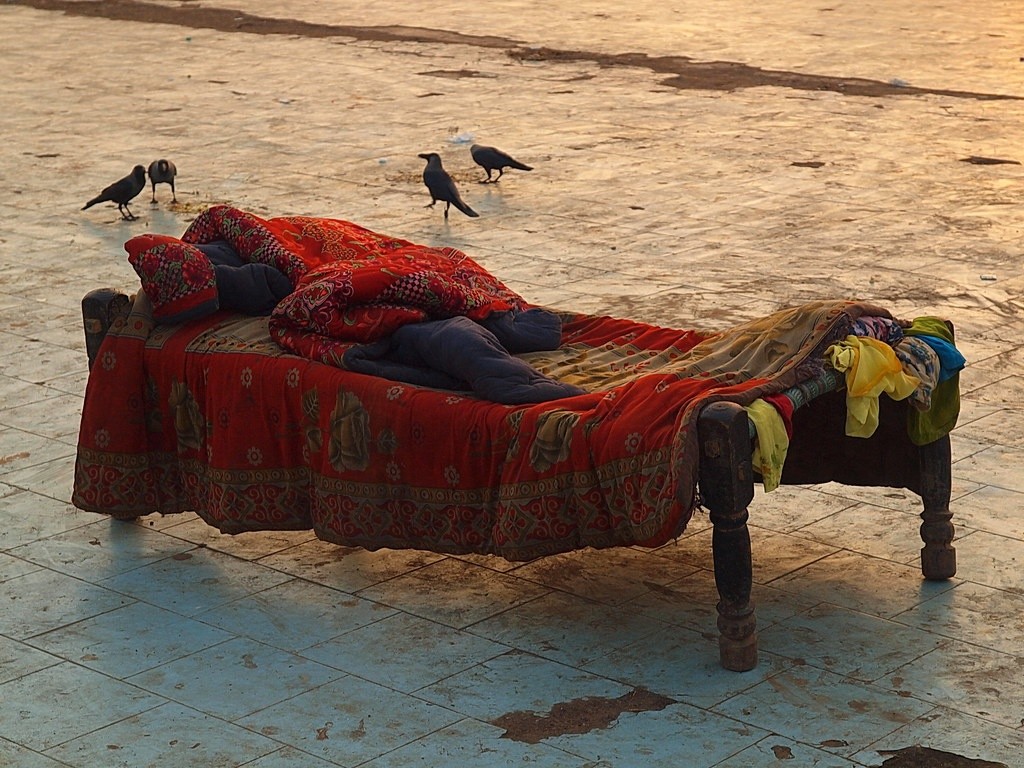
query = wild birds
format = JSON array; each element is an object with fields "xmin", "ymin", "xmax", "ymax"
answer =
[
  {"xmin": 81, "ymin": 159, "xmax": 177, "ymax": 219},
  {"xmin": 470, "ymin": 144, "xmax": 534, "ymax": 185},
  {"xmin": 417, "ymin": 153, "xmax": 481, "ymax": 220}
]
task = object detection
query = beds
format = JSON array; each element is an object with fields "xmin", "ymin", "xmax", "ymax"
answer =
[{"xmin": 69, "ymin": 205, "xmax": 967, "ymax": 674}]
